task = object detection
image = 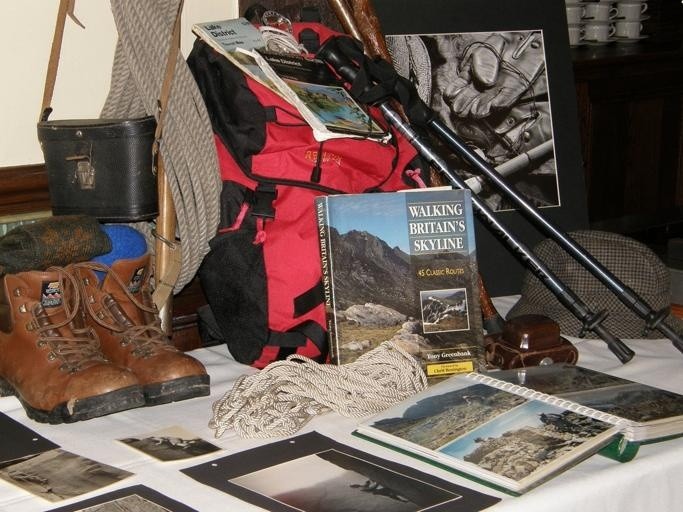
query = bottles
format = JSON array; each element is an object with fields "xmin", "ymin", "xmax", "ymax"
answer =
[{"xmin": 505, "ymin": 231, "xmax": 682, "ymax": 340}]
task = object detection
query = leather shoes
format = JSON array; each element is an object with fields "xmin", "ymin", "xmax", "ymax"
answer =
[{"xmin": 444, "ymin": 32, "xmax": 546, "ymax": 121}]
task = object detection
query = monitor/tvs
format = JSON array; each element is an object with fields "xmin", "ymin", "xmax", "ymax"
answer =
[
  {"xmin": 191, "ymin": 17, "xmax": 392, "ymax": 142},
  {"xmin": 351, "ymin": 363, "xmax": 683, "ymax": 497},
  {"xmin": 315, "ymin": 186, "xmax": 487, "ymax": 387}
]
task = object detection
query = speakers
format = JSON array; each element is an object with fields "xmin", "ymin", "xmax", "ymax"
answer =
[{"xmin": 1, "ymin": 251, "xmax": 210, "ymax": 425}]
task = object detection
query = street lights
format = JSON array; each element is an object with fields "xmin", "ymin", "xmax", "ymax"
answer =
[{"xmin": 38, "ymin": 114, "xmax": 159, "ymax": 221}]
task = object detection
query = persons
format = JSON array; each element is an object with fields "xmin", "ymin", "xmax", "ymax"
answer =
[
  {"xmin": 537, "ymin": 412, "xmax": 582, "ymax": 434},
  {"xmin": 462, "ymin": 395, "xmax": 512, "ymax": 410}
]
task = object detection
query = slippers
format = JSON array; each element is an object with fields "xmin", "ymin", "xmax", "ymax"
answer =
[{"xmin": 186, "ymin": 4, "xmax": 432, "ymax": 370}]
